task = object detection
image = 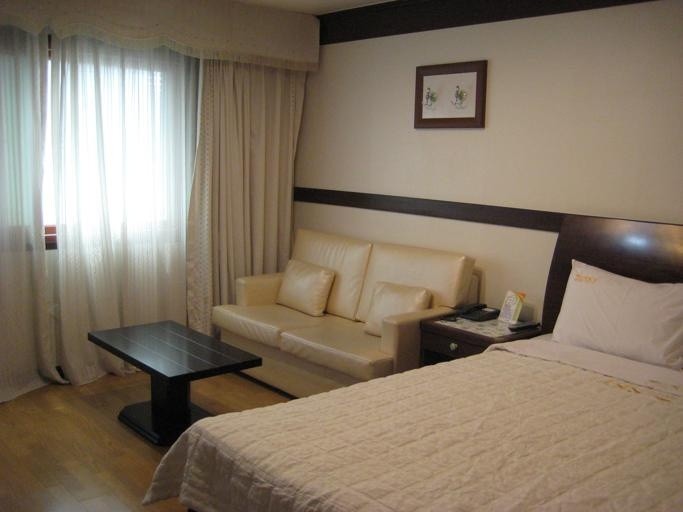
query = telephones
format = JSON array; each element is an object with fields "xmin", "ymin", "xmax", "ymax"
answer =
[{"xmin": 458, "ymin": 304, "xmax": 500, "ymax": 322}]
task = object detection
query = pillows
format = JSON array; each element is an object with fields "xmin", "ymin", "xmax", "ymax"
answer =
[
  {"xmin": 290, "ymin": 228, "xmax": 372, "ymax": 321},
  {"xmin": 354, "ymin": 242, "xmax": 476, "ymax": 324},
  {"xmin": 362, "ymin": 280, "xmax": 433, "ymax": 337},
  {"xmin": 275, "ymin": 259, "xmax": 336, "ymax": 317},
  {"xmin": 551, "ymin": 258, "xmax": 682, "ymax": 372}
]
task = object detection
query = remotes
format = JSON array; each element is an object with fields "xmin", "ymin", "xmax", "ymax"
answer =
[{"xmin": 508, "ymin": 320, "xmax": 540, "ymax": 332}]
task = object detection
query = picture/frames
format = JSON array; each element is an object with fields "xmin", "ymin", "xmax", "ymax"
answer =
[{"xmin": 413, "ymin": 60, "xmax": 488, "ymax": 129}]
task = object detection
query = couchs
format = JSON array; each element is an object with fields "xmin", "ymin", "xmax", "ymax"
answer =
[{"xmin": 210, "ymin": 274, "xmax": 479, "ymax": 398}]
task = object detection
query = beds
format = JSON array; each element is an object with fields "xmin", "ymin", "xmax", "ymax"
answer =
[{"xmin": 140, "ymin": 213, "xmax": 683, "ymax": 512}]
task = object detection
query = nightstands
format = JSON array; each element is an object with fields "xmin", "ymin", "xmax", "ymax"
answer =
[{"xmin": 419, "ymin": 315, "xmax": 549, "ymax": 366}]
